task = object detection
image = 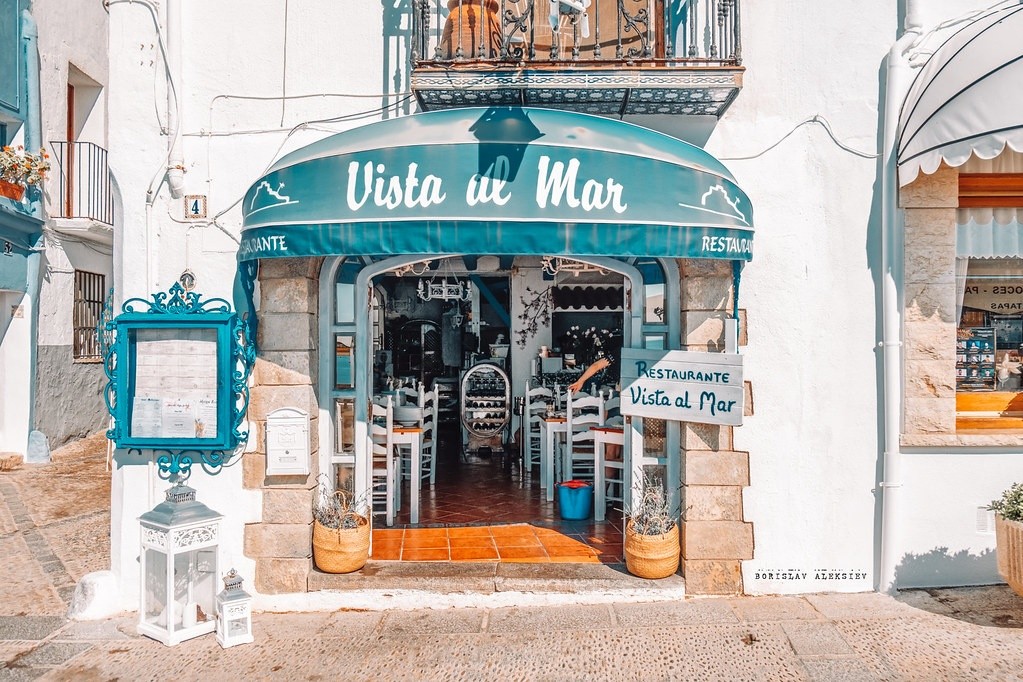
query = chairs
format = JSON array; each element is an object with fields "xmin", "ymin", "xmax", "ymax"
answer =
[
  {"xmin": 373, "ymin": 376, "xmax": 438, "ymax": 528},
  {"xmin": 521, "ymin": 379, "xmax": 613, "ymax": 500}
]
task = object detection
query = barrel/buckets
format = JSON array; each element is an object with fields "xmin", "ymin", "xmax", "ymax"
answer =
[{"xmin": 556, "ymin": 484, "xmax": 593, "ymax": 521}]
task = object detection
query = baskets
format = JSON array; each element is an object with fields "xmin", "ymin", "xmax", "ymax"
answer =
[
  {"xmin": 625, "ymin": 517, "xmax": 680, "ymax": 579},
  {"xmin": 313, "ymin": 512, "xmax": 371, "ymax": 574}
]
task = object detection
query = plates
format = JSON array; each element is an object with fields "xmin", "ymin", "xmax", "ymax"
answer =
[{"xmin": 398, "ymin": 422, "xmax": 417, "ymax": 427}]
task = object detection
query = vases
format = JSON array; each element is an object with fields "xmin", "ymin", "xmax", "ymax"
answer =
[{"xmin": 0, "ymin": 179, "xmax": 25, "ymax": 200}]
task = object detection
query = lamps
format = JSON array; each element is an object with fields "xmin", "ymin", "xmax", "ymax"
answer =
[
  {"xmin": 541, "ymin": 255, "xmax": 615, "ymax": 277},
  {"xmin": 386, "ymin": 260, "xmax": 430, "ymax": 278},
  {"xmin": 417, "ymin": 259, "xmax": 472, "ymax": 302}
]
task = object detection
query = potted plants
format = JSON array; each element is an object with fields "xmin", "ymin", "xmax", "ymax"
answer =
[
  {"xmin": 313, "ymin": 474, "xmax": 372, "ymax": 574},
  {"xmin": 981, "ymin": 482, "xmax": 1023, "ymax": 598},
  {"xmin": 614, "ymin": 466, "xmax": 691, "ymax": 580}
]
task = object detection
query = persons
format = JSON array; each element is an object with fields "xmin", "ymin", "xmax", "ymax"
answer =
[{"xmin": 568, "ymin": 345, "xmax": 623, "ymax": 507}]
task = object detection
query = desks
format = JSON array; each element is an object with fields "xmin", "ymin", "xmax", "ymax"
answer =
[
  {"xmin": 377, "ymin": 423, "xmax": 424, "ymax": 525},
  {"xmin": 590, "ymin": 423, "xmax": 632, "ymax": 520},
  {"xmin": 536, "ymin": 410, "xmax": 592, "ymax": 503}
]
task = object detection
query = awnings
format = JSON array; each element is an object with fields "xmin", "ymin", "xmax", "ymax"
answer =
[
  {"xmin": 895, "ymin": 0, "xmax": 1023, "ymax": 191},
  {"xmin": 236, "ymin": 105, "xmax": 707, "ymax": 262}
]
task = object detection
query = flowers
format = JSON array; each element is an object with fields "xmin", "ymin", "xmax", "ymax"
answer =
[{"xmin": 0, "ymin": 145, "xmax": 50, "ymax": 184}]
